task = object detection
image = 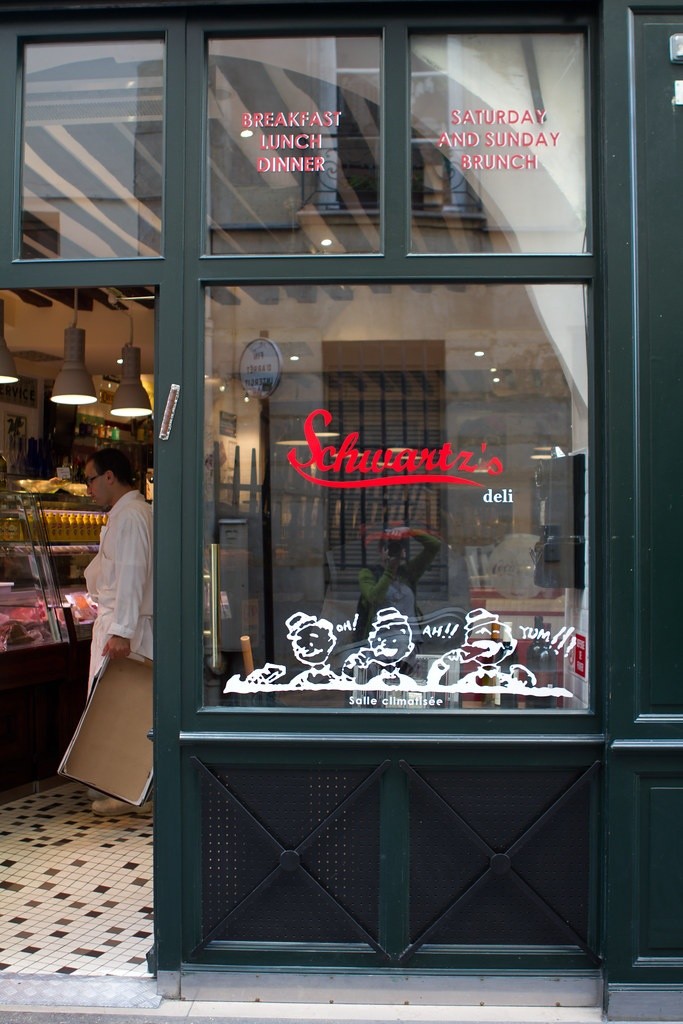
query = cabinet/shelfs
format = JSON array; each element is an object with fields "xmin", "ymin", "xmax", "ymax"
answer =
[
  {"xmin": 0, "ymin": 488, "xmax": 110, "ymax": 789},
  {"xmin": 55, "ymin": 396, "xmax": 143, "ymax": 489}
]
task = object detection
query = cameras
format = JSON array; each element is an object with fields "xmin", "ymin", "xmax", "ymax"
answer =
[{"xmin": 387, "ymin": 539, "xmax": 402, "ymax": 557}]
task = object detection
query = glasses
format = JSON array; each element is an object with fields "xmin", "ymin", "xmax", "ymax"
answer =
[{"xmin": 85, "ymin": 471, "xmax": 107, "ymax": 487}]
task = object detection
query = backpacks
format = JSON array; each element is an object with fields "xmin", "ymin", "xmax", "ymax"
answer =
[{"xmin": 353, "ymin": 565, "xmax": 382, "ymax": 645}]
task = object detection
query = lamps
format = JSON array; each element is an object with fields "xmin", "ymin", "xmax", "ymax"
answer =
[
  {"xmin": 0, "ymin": 298, "xmax": 19, "ymax": 384},
  {"xmin": 51, "ymin": 288, "xmax": 99, "ymax": 405},
  {"xmin": 110, "ymin": 299, "xmax": 152, "ymax": 418}
]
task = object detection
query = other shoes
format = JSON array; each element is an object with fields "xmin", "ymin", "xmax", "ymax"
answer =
[
  {"xmin": 91, "ymin": 795, "xmax": 152, "ymax": 815},
  {"xmin": 88, "ymin": 787, "xmax": 106, "ymax": 800}
]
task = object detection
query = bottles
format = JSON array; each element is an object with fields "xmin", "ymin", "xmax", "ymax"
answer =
[
  {"xmin": 0, "ymin": 508, "xmax": 108, "ymax": 542},
  {"xmin": 525, "ymin": 615, "xmax": 557, "ymax": 708},
  {"xmin": 137, "ymin": 415, "xmax": 154, "ymax": 446},
  {"xmin": 480, "ymin": 621, "xmax": 521, "ymax": 709},
  {"xmin": 77, "ymin": 420, "xmax": 120, "ymax": 441}
]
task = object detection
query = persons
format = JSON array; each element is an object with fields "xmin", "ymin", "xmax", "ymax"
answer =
[
  {"xmin": 359, "ymin": 526, "xmax": 442, "ymax": 675},
  {"xmin": 83, "ymin": 447, "xmax": 153, "ymax": 813}
]
